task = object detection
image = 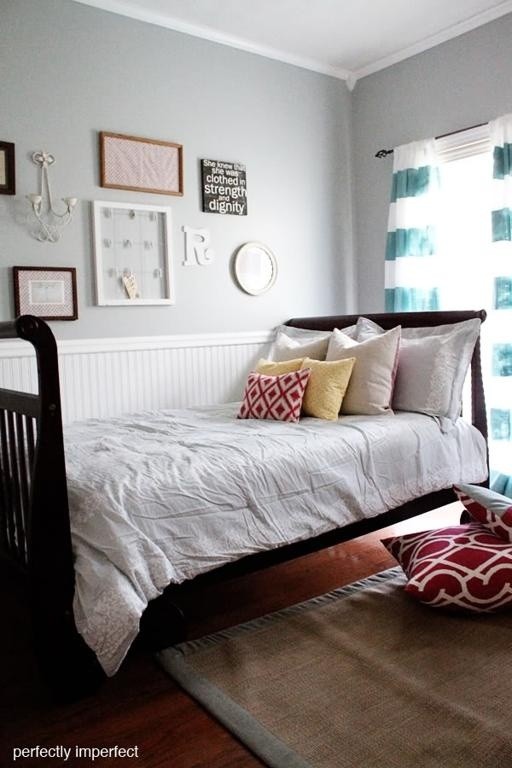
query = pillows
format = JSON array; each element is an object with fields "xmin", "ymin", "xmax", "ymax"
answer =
[
  {"xmin": 452, "ymin": 483, "xmax": 511, "ymax": 544},
  {"xmin": 269, "ymin": 323, "xmax": 332, "ymax": 361},
  {"xmin": 254, "ymin": 357, "xmax": 304, "ymax": 377},
  {"xmin": 325, "ymin": 324, "xmax": 402, "ymax": 417},
  {"xmin": 300, "ymin": 356, "xmax": 355, "ymax": 421},
  {"xmin": 353, "ymin": 315, "xmax": 482, "ymax": 436},
  {"xmin": 236, "ymin": 367, "xmax": 311, "ymax": 424},
  {"xmin": 379, "ymin": 520, "xmax": 512, "ymax": 616}
]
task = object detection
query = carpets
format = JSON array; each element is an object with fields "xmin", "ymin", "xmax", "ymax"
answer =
[{"xmin": 159, "ymin": 568, "xmax": 512, "ymax": 767}]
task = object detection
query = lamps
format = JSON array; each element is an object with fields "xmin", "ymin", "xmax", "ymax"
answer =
[{"xmin": 25, "ymin": 150, "xmax": 77, "ymax": 243}]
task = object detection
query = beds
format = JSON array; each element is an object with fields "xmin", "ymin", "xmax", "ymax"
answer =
[{"xmin": 0, "ymin": 309, "xmax": 496, "ymax": 703}]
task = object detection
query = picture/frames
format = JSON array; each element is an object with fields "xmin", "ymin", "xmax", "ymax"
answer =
[
  {"xmin": 0, "ymin": 140, "xmax": 16, "ymax": 196},
  {"xmin": 99, "ymin": 129, "xmax": 184, "ymax": 197},
  {"xmin": 12, "ymin": 265, "xmax": 78, "ymax": 321},
  {"xmin": 90, "ymin": 199, "xmax": 173, "ymax": 306}
]
